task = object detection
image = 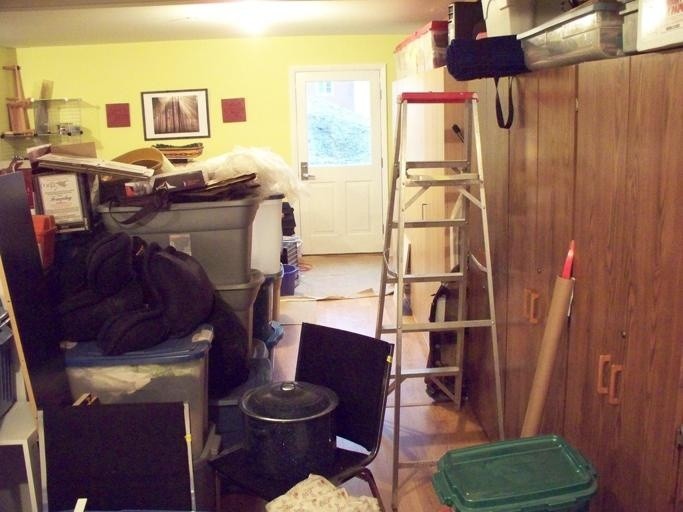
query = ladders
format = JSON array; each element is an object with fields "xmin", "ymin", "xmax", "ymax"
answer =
[{"xmin": 375, "ymin": 92, "xmax": 505, "ymax": 512}]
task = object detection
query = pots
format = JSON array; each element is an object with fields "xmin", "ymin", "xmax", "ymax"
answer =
[{"xmin": 238, "ymin": 380, "xmax": 340, "ymax": 480}]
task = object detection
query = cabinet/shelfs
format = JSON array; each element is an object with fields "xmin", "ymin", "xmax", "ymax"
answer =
[
  {"xmin": 505, "ymin": 69, "xmax": 577, "ymax": 440},
  {"xmin": 458, "ymin": 79, "xmax": 509, "ymax": 441},
  {"xmin": 387, "ymin": 68, "xmax": 465, "ymax": 349},
  {"xmin": 563, "ymin": 57, "xmax": 682, "ymax": 512}
]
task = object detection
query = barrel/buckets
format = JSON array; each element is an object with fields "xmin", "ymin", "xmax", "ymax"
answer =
[{"xmin": 280, "ymin": 264, "xmax": 298, "ymax": 296}]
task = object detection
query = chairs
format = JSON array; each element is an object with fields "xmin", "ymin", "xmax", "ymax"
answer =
[{"xmin": 210, "ymin": 322, "xmax": 395, "ymax": 512}]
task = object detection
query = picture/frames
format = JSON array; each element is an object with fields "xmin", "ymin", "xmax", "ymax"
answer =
[{"xmin": 141, "ymin": 89, "xmax": 211, "ymax": 140}]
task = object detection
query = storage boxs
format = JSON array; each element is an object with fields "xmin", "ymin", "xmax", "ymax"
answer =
[
  {"xmin": 517, "ymin": 0, "xmax": 621, "ymax": 70},
  {"xmin": 392, "ymin": 20, "xmax": 449, "ymax": 79},
  {"xmin": 208, "ymin": 357, "xmax": 272, "ymax": 437},
  {"xmin": 212, "ymin": 269, "xmax": 267, "ymax": 371},
  {"xmin": 482, "ymin": 0, "xmax": 571, "ymax": 37},
  {"xmin": 185, "ymin": 423, "xmax": 223, "ymax": 511},
  {"xmin": 60, "ymin": 324, "xmax": 215, "ymax": 456},
  {"xmin": 98, "ymin": 198, "xmax": 263, "ymax": 283}
]
